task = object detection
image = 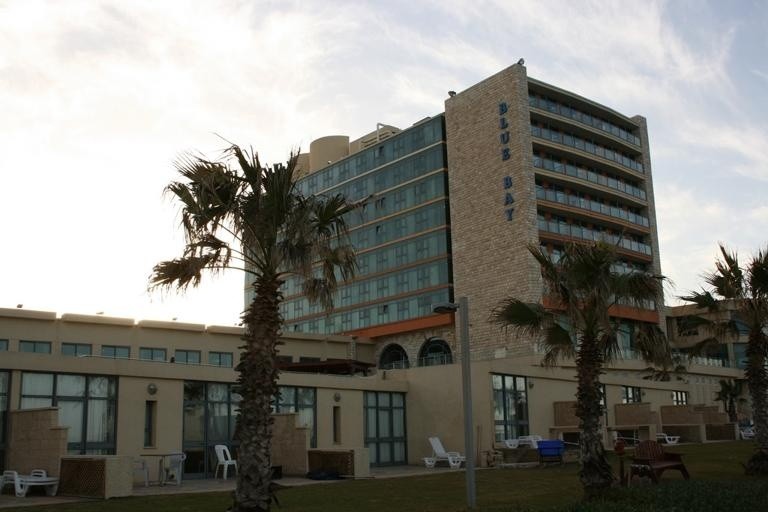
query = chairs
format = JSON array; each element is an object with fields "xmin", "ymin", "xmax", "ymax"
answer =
[
  {"xmin": 1, "ymin": 469, "xmax": 60, "ymax": 498},
  {"xmin": 504, "ymin": 435, "xmax": 542, "ymax": 450},
  {"xmin": 214, "ymin": 444, "xmax": 238, "ymax": 481},
  {"xmin": 421, "ymin": 437, "xmax": 467, "ymax": 469},
  {"xmin": 743, "ymin": 429, "xmax": 755, "ymax": 437},
  {"xmin": 629, "ymin": 439, "xmax": 689, "ymax": 488},
  {"xmin": 656, "ymin": 433, "xmax": 680, "ymax": 443}
]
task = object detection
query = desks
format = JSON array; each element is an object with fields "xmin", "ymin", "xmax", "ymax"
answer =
[{"xmin": 139, "ymin": 452, "xmax": 186, "ymax": 487}]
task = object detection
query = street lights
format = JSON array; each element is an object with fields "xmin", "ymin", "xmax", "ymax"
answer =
[{"xmin": 434, "ymin": 296, "xmax": 477, "ymax": 511}]
